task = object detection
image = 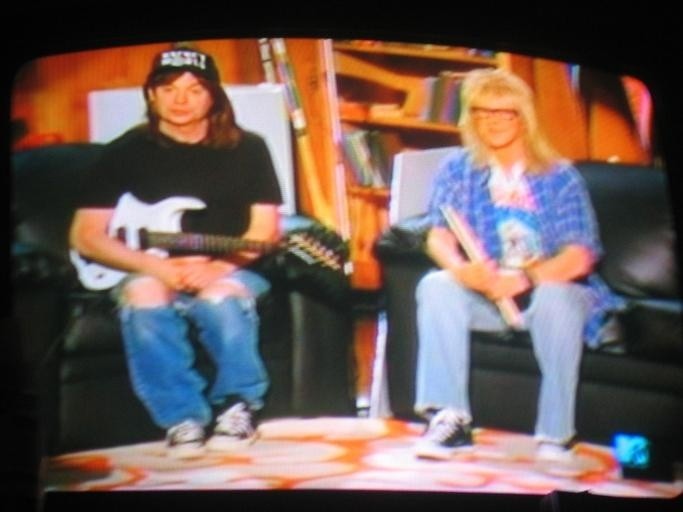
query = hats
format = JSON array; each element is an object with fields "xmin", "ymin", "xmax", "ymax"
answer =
[{"xmin": 147, "ymin": 46, "xmax": 217, "ymax": 80}]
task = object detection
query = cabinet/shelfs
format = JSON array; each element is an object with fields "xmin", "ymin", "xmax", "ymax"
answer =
[{"xmin": 276, "ymin": 37, "xmax": 512, "ymax": 291}]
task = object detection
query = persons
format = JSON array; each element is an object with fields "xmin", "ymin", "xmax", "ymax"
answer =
[
  {"xmin": 410, "ymin": 64, "xmax": 621, "ymax": 476},
  {"xmin": 64, "ymin": 45, "xmax": 281, "ymax": 464}
]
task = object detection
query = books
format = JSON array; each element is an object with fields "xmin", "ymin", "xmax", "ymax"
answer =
[
  {"xmin": 333, "ymin": 69, "xmax": 482, "ymax": 192},
  {"xmin": 327, "ymin": 37, "xmax": 494, "ymax": 247}
]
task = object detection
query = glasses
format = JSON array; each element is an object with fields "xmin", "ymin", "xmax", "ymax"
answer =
[{"xmin": 469, "ymin": 106, "xmax": 517, "ymax": 121}]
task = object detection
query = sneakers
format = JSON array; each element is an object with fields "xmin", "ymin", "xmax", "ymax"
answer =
[
  {"xmin": 412, "ymin": 408, "xmax": 480, "ymax": 461},
  {"xmin": 203, "ymin": 401, "xmax": 262, "ymax": 452},
  {"xmin": 166, "ymin": 419, "xmax": 206, "ymax": 453},
  {"xmin": 538, "ymin": 442, "xmax": 572, "ymax": 461}
]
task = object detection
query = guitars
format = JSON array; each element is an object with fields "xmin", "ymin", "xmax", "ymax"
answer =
[{"xmin": 69, "ymin": 192, "xmax": 346, "ymax": 292}]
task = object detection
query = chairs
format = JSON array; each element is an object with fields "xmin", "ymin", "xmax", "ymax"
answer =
[
  {"xmin": 369, "ymin": 161, "xmax": 683, "ymax": 483},
  {"xmin": 0, "ymin": 144, "xmax": 358, "ymax": 454}
]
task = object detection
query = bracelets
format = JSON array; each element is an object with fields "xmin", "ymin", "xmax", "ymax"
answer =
[{"xmin": 520, "ymin": 267, "xmax": 534, "ymax": 291}]
